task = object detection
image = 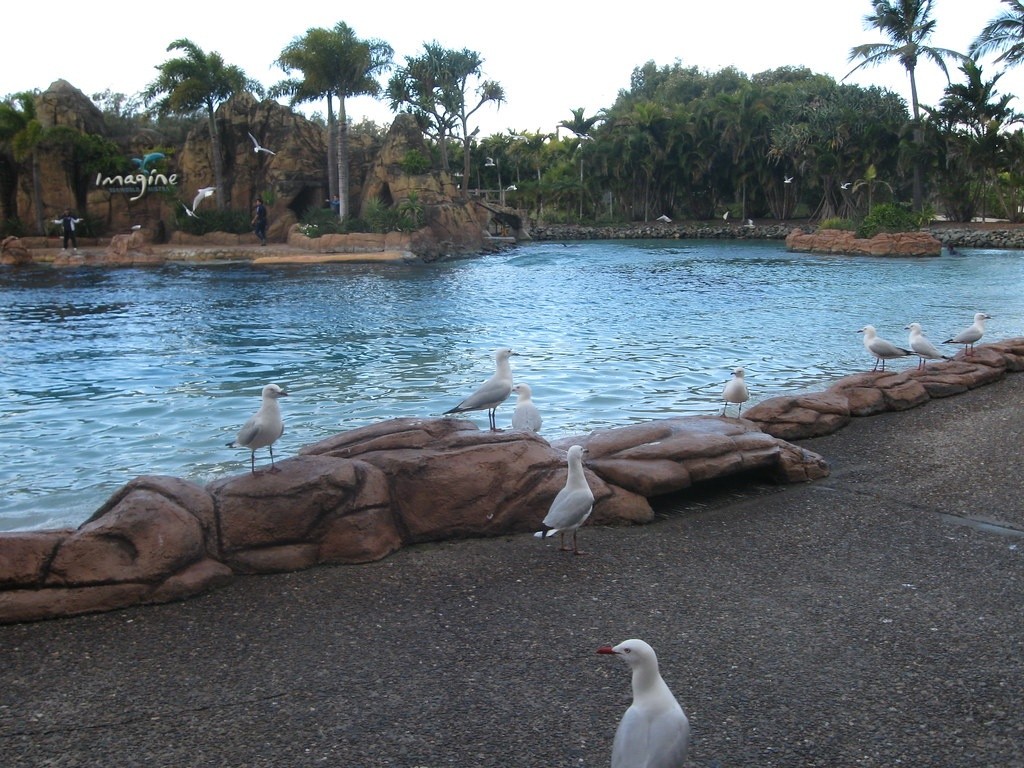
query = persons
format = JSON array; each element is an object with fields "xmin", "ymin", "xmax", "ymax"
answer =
[
  {"xmin": 324, "ymin": 195, "xmax": 340, "ymax": 215},
  {"xmin": 49, "ymin": 209, "xmax": 85, "ymax": 252},
  {"xmin": 947, "ymin": 245, "xmax": 965, "ymax": 257},
  {"xmin": 252, "ymin": 197, "xmax": 268, "ymax": 246}
]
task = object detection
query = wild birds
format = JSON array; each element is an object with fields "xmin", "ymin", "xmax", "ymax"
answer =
[
  {"xmin": 533, "ymin": 444, "xmax": 596, "ymax": 556},
  {"xmin": 597, "ymin": 639, "xmax": 690, "ymax": 768},
  {"xmin": 857, "ymin": 325, "xmax": 919, "ymax": 373},
  {"xmin": 485, "ymin": 157, "xmax": 496, "ymax": 166},
  {"xmin": 178, "ymin": 186, "xmax": 217, "ymax": 218},
  {"xmin": 783, "ymin": 175, "xmax": 793, "ymax": 183},
  {"xmin": 941, "ymin": 313, "xmax": 992, "ymax": 358},
  {"xmin": 512, "ymin": 383, "xmax": 542, "ymax": 432},
  {"xmin": 656, "ymin": 214, "xmax": 672, "ymax": 222},
  {"xmin": 840, "ymin": 181, "xmax": 852, "ymax": 189},
  {"xmin": 248, "ymin": 131, "xmax": 276, "ymax": 156},
  {"xmin": 904, "ymin": 323, "xmax": 955, "ymax": 372},
  {"xmin": 506, "ymin": 185, "xmax": 517, "ymax": 191},
  {"xmin": 226, "ymin": 384, "xmax": 289, "ymax": 480},
  {"xmin": 719, "ymin": 367, "xmax": 750, "ymax": 419},
  {"xmin": 442, "ymin": 348, "xmax": 522, "ymax": 433},
  {"xmin": 510, "ymin": 135, "xmax": 528, "ymax": 143}
]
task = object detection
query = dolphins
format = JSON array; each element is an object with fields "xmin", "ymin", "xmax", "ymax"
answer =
[{"xmin": 131, "ymin": 152, "xmax": 165, "ymax": 174}]
